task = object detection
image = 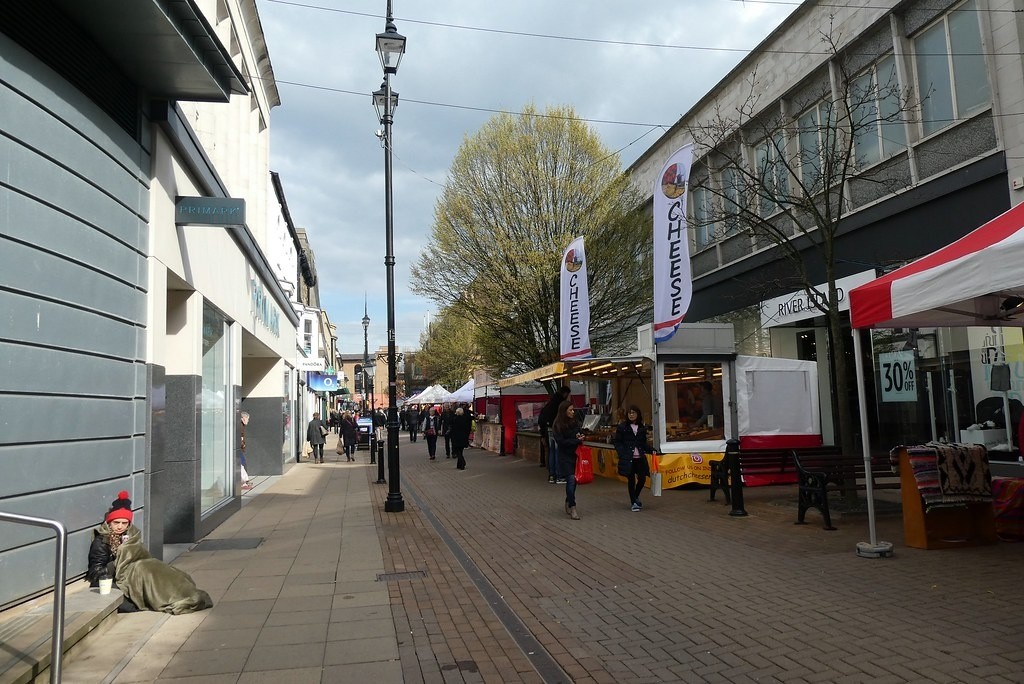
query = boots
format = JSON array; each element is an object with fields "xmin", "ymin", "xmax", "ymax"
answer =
[
  {"xmin": 347, "ymin": 456, "xmax": 350, "ymax": 462},
  {"xmin": 320, "ymin": 456, "xmax": 324, "ymax": 463},
  {"xmin": 351, "ymin": 453, "xmax": 355, "ymax": 462},
  {"xmin": 315, "ymin": 458, "xmax": 319, "ymax": 464}
]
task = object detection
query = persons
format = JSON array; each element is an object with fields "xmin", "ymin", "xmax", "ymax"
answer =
[
  {"xmin": 552, "ymin": 401, "xmax": 584, "ymax": 520},
  {"xmin": 688, "ymin": 381, "xmax": 720, "ymax": 428},
  {"xmin": 677, "ymin": 386, "xmax": 694, "ymax": 416},
  {"xmin": 241, "ymin": 412, "xmax": 253, "ymax": 489},
  {"xmin": 307, "ymin": 412, "xmax": 328, "ymax": 463},
  {"xmin": 339, "ymin": 410, "xmax": 360, "ymax": 461},
  {"xmin": 374, "ymin": 408, "xmax": 385, "ymax": 441},
  {"xmin": 368, "ymin": 407, "xmax": 387, "ymax": 417},
  {"xmin": 398, "ymin": 405, "xmax": 431, "ymax": 442},
  {"xmin": 422, "ymin": 408, "xmax": 441, "ymax": 460},
  {"xmin": 329, "ymin": 408, "xmax": 363, "ymax": 434},
  {"xmin": 614, "ymin": 406, "xmax": 657, "ymax": 512},
  {"xmin": 538, "ymin": 386, "xmax": 571, "ymax": 484},
  {"xmin": 87, "ymin": 492, "xmax": 213, "ymax": 613},
  {"xmin": 438, "ymin": 402, "xmax": 478, "ymax": 470}
]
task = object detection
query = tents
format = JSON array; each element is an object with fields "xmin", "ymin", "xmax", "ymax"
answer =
[
  {"xmin": 850, "ymin": 201, "xmax": 1024, "ymax": 557},
  {"xmin": 442, "ymin": 380, "xmax": 473, "ymax": 403},
  {"xmin": 409, "ymin": 384, "xmax": 450, "ymax": 407}
]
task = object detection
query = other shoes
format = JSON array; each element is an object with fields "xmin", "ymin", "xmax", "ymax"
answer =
[
  {"xmin": 432, "ymin": 455, "xmax": 435, "ymax": 459},
  {"xmin": 447, "ymin": 455, "xmax": 450, "ymax": 458},
  {"xmin": 430, "ymin": 456, "xmax": 432, "ymax": 459},
  {"xmin": 242, "ymin": 484, "xmax": 252, "ymax": 489},
  {"xmin": 546, "ymin": 465, "xmax": 550, "ymax": 470},
  {"xmin": 540, "ymin": 464, "xmax": 546, "ymax": 467},
  {"xmin": 452, "ymin": 454, "xmax": 456, "ymax": 458}
]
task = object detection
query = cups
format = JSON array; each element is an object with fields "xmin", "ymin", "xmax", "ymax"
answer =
[
  {"xmin": 478, "ymin": 415, "xmax": 485, "ymax": 419},
  {"xmin": 99, "ymin": 575, "xmax": 113, "ymax": 595}
]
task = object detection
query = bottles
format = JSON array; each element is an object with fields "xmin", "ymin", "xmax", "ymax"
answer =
[{"xmin": 495, "ymin": 414, "xmax": 499, "ymax": 423}]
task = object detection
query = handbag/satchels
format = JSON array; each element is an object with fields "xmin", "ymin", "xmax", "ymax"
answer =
[
  {"xmin": 336, "ymin": 438, "xmax": 345, "ymax": 455},
  {"xmin": 465, "ymin": 441, "xmax": 469, "ymax": 448},
  {"xmin": 427, "ymin": 428, "xmax": 433, "ymax": 436},
  {"xmin": 320, "ymin": 425, "xmax": 328, "ymax": 436},
  {"xmin": 652, "ymin": 454, "xmax": 662, "ymax": 496},
  {"xmin": 575, "ymin": 436, "xmax": 594, "ymax": 485},
  {"xmin": 356, "ymin": 431, "xmax": 361, "ymax": 441}
]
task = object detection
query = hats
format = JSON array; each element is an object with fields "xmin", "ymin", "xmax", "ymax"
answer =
[{"xmin": 107, "ymin": 491, "xmax": 133, "ymax": 523}]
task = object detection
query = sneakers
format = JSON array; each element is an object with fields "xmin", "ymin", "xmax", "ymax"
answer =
[
  {"xmin": 633, "ymin": 496, "xmax": 643, "ymax": 508},
  {"xmin": 549, "ymin": 476, "xmax": 555, "ymax": 483},
  {"xmin": 556, "ymin": 477, "xmax": 568, "ymax": 484},
  {"xmin": 631, "ymin": 504, "xmax": 639, "ymax": 511}
]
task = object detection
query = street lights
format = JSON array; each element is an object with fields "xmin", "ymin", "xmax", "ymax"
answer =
[
  {"xmin": 370, "ymin": 2, "xmax": 405, "ymax": 513},
  {"xmin": 362, "ymin": 302, "xmax": 371, "ymax": 418}
]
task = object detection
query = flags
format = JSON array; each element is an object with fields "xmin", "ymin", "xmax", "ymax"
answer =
[
  {"xmin": 560, "ymin": 235, "xmax": 592, "ymax": 361},
  {"xmin": 653, "ymin": 141, "xmax": 694, "ymax": 343}
]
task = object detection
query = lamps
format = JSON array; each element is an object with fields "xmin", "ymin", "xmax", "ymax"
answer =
[{"xmin": 1000, "ymin": 296, "xmax": 1024, "ymax": 311}]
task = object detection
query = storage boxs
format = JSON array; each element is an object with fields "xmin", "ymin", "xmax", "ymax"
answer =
[{"xmin": 960, "ymin": 429, "xmax": 1007, "ymax": 450}]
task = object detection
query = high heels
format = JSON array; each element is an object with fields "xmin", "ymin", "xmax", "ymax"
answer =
[
  {"xmin": 569, "ymin": 506, "xmax": 580, "ymax": 520},
  {"xmin": 565, "ymin": 500, "xmax": 571, "ymax": 514}
]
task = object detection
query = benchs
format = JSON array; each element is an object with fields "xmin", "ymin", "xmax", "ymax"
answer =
[
  {"xmin": 709, "ymin": 438, "xmax": 845, "ymax": 517},
  {"xmin": 791, "ymin": 450, "xmax": 901, "ymax": 531}
]
